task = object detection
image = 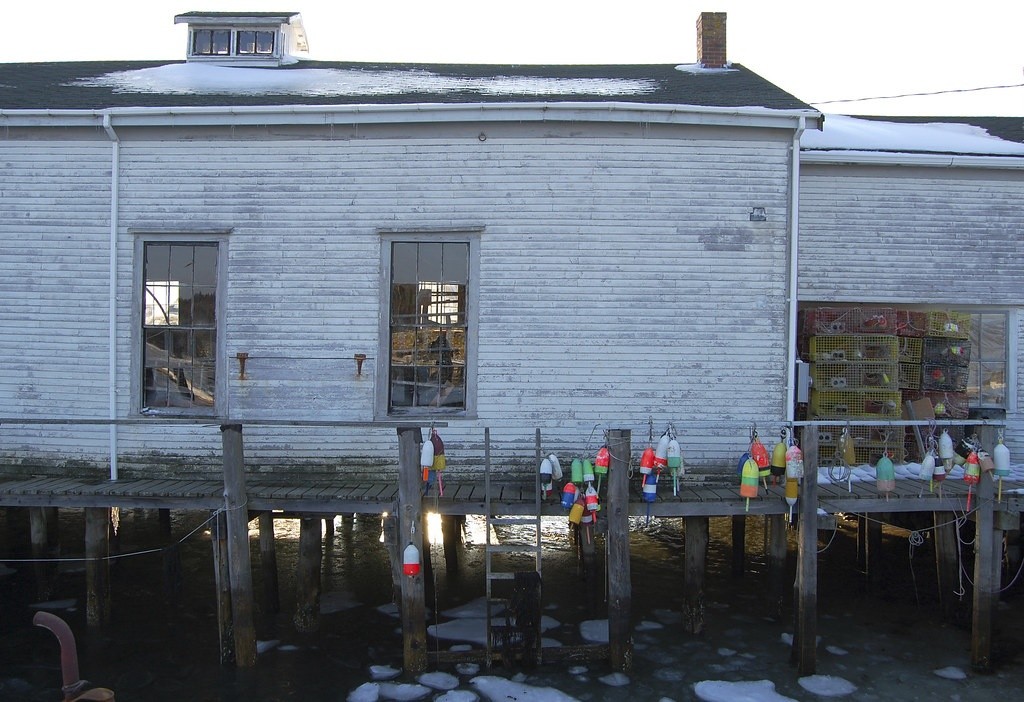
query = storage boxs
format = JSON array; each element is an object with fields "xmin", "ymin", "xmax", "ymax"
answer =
[{"xmin": 798, "ymin": 308, "xmax": 971, "ymax": 470}]
